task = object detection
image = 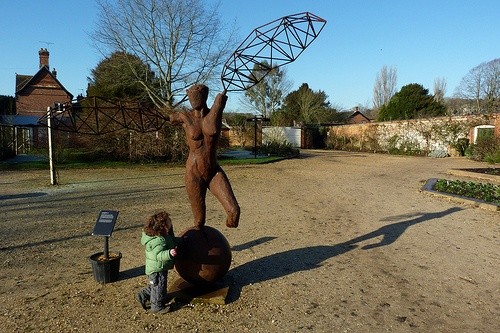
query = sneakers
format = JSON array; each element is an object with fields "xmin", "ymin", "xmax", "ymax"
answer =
[
  {"xmin": 152, "ymin": 306, "xmax": 169, "ymax": 313},
  {"xmin": 137, "ymin": 292, "xmax": 147, "ymax": 312}
]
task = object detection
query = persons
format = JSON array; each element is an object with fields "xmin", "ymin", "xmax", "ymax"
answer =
[
  {"xmin": 134, "ymin": 211, "xmax": 177, "ymax": 314},
  {"xmin": 170, "ymin": 84, "xmax": 240, "ymax": 230}
]
